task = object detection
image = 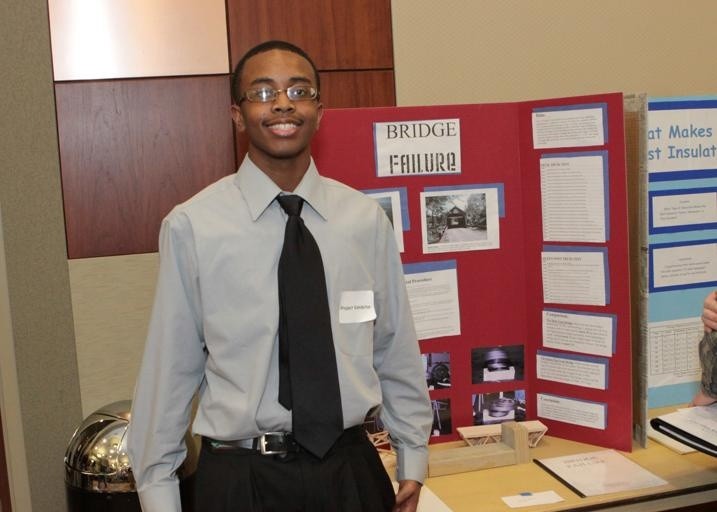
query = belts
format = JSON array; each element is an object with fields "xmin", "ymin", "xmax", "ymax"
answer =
[{"xmin": 200, "ymin": 422, "xmax": 367, "ymax": 459}]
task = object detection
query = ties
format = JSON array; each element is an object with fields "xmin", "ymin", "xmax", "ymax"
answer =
[{"xmin": 272, "ymin": 195, "xmax": 345, "ymax": 459}]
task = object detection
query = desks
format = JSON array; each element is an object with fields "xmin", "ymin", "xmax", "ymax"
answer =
[{"xmin": 378, "ymin": 406, "xmax": 717, "ymax": 512}]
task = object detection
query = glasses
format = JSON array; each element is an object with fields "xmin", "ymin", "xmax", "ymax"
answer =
[{"xmin": 234, "ymin": 85, "xmax": 320, "ymax": 105}]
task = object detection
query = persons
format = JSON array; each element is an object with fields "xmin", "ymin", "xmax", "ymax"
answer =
[
  {"xmin": 695, "ymin": 289, "xmax": 716, "ymax": 407},
  {"xmin": 127, "ymin": 40, "xmax": 433, "ymax": 511}
]
